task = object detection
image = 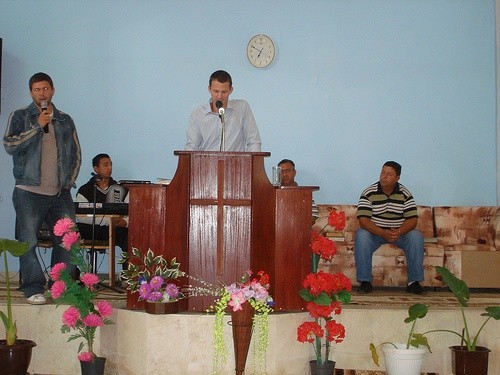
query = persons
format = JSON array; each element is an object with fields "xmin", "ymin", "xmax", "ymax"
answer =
[
  {"xmin": 76, "ymin": 153, "xmax": 129, "ymax": 284},
  {"xmin": 184, "ymin": 69, "xmax": 262, "ymax": 152},
  {"xmin": 354, "ymin": 161, "xmax": 427, "ymax": 295},
  {"xmin": 278, "ymin": 159, "xmax": 319, "ymax": 226},
  {"xmin": 2, "ymin": 72, "xmax": 82, "ymax": 305}
]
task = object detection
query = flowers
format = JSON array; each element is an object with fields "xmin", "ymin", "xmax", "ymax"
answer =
[
  {"xmin": 47, "ymin": 217, "xmax": 115, "ymax": 362},
  {"xmin": 115, "ymin": 244, "xmax": 186, "ymax": 302},
  {"xmin": 295, "ymin": 208, "xmax": 352, "ymax": 364},
  {"xmin": 184, "ymin": 269, "xmax": 275, "ymax": 375}
]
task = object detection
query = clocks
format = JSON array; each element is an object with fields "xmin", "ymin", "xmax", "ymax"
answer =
[{"xmin": 245, "ymin": 32, "xmax": 277, "ymax": 70}]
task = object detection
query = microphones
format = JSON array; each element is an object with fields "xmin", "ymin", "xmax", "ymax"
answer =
[
  {"xmin": 216, "ymin": 100, "xmax": 223, "ymax": 121},
  {"xmin": 41, "ymin": 99, "xmax": 50, "ymax": 133},
  {"xmin": 91, "ymin": 172, "xmax": 102, "ymax": 180}
]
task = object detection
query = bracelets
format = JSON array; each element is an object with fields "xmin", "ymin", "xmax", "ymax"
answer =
[{"xmin": 397, "ymin": 228, "xmax": 401, "ymax": 238}]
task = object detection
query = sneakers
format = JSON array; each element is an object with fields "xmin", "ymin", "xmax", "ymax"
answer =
[
  {"xmin": 357, "ymin": 282, "xmax": 371, "ymax": 294},
  {"xmin": 407, "ymin": 282, "xmax": 425, "ymax": 293},
  {"xmin": 26, "ymin": 294, "xmax": 46, "ymax": 305}
]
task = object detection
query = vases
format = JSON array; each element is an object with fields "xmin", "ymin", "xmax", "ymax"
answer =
[
  {"xmin": 80, "ymin": 357, "xmax": 107, "ymax": 375},
  {"xmin": 145, "ymin": 302, "xmax": 178, "ymax": 314},
  {"xmin": 309, "ymin": 359, "xmax": 336, "ymax": 375},
  {"xmin": 228, "ymin": 304, "xmax": 256, "ymax": 375}
]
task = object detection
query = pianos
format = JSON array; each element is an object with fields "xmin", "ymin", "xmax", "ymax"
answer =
[{"xmin": 72, "ymin": 201, "xmax": 129, "ymax": 295}]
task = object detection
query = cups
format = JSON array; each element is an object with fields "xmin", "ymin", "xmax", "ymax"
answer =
[{"xmin": 272, "ymin": 166, "xmax": 282, "ymax": 189}]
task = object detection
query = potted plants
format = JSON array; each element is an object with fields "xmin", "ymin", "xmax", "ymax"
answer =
[
  {"xmin": 368, "ymin": 266, "xmax": 500, "ymax": 375},
  {"xmin": 0, "ymin": 236, "xmax": 38, "ymax": 375}
]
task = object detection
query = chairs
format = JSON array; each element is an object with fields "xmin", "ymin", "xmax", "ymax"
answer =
[{"xmin": 13, "ymin": 215, "xmax": 109, "ymax": 290}]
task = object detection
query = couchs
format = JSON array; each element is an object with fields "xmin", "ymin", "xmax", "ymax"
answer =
[{"xmin": 318, "ymin": 204, "xmax": 500, "ymax": 288}]
